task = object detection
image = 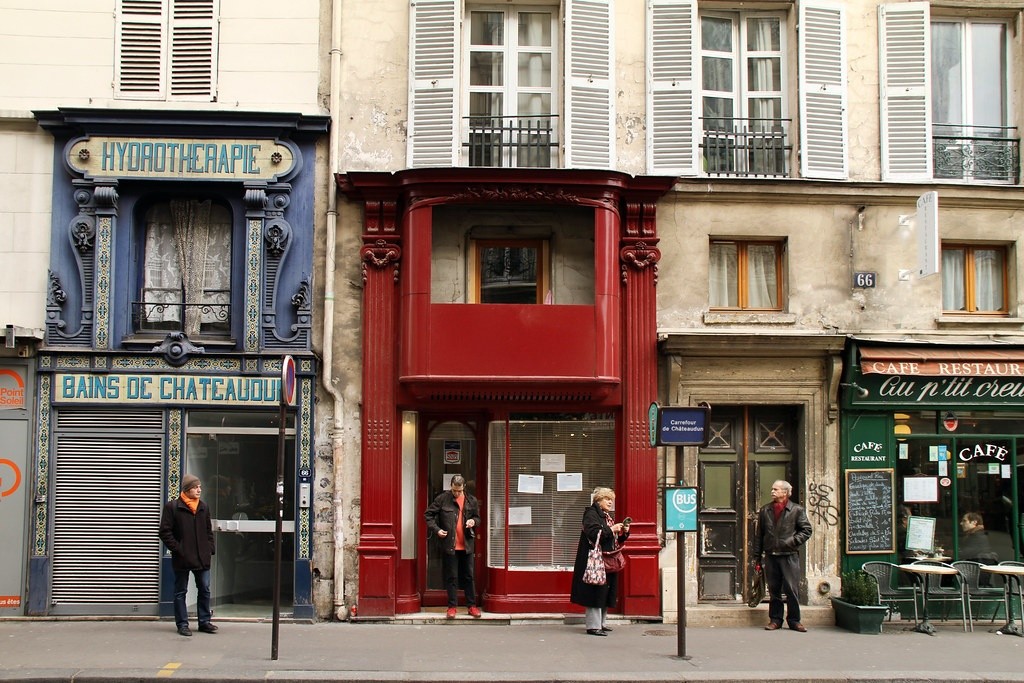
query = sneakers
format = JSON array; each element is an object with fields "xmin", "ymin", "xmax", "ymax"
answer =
[
  {"xmin": 468, "ymin": 606, "xmax": 481, "ymax": 617},
  {"xmin": 447, "ymin": 608, "xmax": 456, "ymax": 618}
]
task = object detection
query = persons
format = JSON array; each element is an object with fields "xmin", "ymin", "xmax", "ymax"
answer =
[
  {"xmin": 571, "ymin": 487, "xmax": 630, "ymax": 636},
  {"xmin": 158, "ymin": 474, "xmax": 218, "ymax": 636},
  {"xmin": 425, "ymin": 474, "xmax": 482, "ymax": 619},
  {"xmin": 895, "ymin": 503, "xmax": 995, "ymax": 589},
  {"xmin": 752, "ymin": 481, "xmax": 813, "ymax": 632}
]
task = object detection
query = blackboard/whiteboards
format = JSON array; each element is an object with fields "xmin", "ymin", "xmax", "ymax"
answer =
[{"xmin": 845, "ymin": 468, "xmax": 895, "ymax": 554}]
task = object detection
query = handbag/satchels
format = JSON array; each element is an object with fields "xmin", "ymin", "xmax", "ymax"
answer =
[
  {"xmin": 747, "ymin": 563, "xmax": 765, "ymax": 607},
  {"xmin": 602, "ymin": 545, "xmax": 626, "ymax": 574},
  {"xmin": 583, "ymin": 530, "xmax": 606, "ymax": 586}
]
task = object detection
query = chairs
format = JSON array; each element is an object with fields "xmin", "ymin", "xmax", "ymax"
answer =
[{"xmin": 859, "ymin": 550, "xmax": 1024, "ymax": 640}]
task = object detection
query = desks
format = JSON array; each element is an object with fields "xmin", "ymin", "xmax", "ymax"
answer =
[
  {"xmin": 899, "ymin": 563, "xmax": 957, "ymax": 636},
  {"xmin": 978, "ymin": 563, "xmax": 1024, "ymax": 638},
  {"xmin": 906, "ymin": 554, "xmax": 955, "ymax": 564}
]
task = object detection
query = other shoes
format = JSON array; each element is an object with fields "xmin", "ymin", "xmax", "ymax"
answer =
[
  {"xmin": 790, "ymin": 623, "xmax": 807, "ymax": 632},
  {"xmin": 765, "ymin": 622, "xmax": 781, "ymax": 630},
  {"xmin": 199, "ymin": 623, "xmax": 218, "ymax": 632},
  {"xmin": 178, "ymin": 627, "xmax": 192, "ymax": 636},
  {"xmin": 587, "ymin": 626, "xmax": 612, "ymax": 636}
]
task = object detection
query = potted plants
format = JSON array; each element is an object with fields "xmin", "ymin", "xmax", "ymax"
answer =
[{"xmin": 825, "ymin": 569, "xmax": 888, "ymax": 635}]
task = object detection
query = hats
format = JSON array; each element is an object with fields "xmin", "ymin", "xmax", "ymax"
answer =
[{"xmin": 182, "ymin": 474, "xmax": 200, "ymax": 493}]
